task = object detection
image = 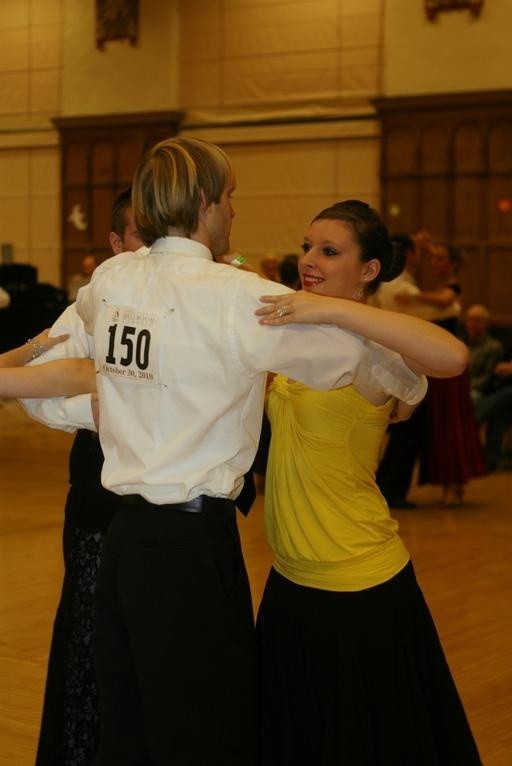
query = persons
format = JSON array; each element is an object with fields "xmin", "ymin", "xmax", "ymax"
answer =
[
  {"xmin": 76, "ymin": 135, "xmax": 428, "ymax": 765},
  {"xmin": 256, "ymin": 200, "xmax": 485, "ymax": 765},
  {"xmin": 0, "ymin": 188, "xmax": 153, "ymax": 766},
  {"xmin": 224, "ymin": 249, "xmax": 304, "ymax": 492},
  {"xmin": 377, "ymin": 232, "xmax": 501, "ymax": 508}
]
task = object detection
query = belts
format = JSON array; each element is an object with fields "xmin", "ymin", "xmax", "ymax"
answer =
[{"xmin": 159, "ymin": 494, "xmax": 204, "ymax": 513}]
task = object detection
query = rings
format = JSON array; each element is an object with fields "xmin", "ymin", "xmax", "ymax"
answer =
[{"xmin": 277, "ymin": 306, "xmax": 284, "ymax": 316}]
